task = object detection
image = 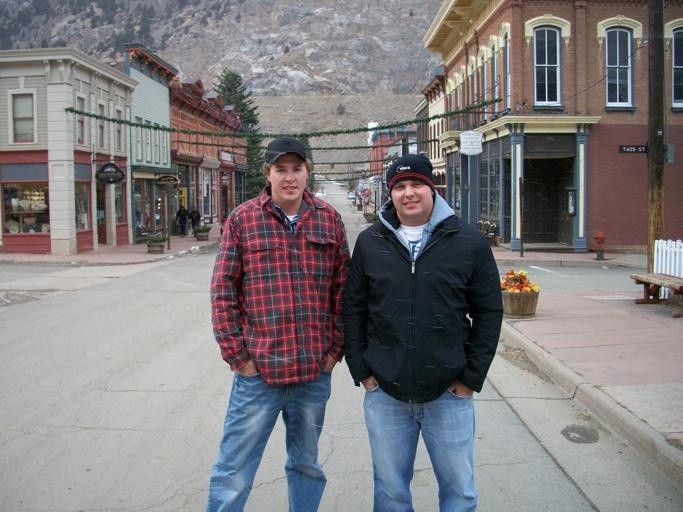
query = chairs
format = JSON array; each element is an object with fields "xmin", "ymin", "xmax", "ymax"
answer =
[{"xmin": 474, "ymin": 220, "xmax": 499, "ymax": 247}]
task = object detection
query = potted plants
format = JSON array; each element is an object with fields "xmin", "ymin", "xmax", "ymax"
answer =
[
  {"xmin": 141, "ymin": 233, "xmax": 169, "ymax": 254},
  {"xmin": 192, "ymin": 224, "xmax": 213, "ymax": 241}
]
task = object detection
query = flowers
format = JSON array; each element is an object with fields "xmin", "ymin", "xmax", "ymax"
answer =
[{"xmin": 500, "ymin": 269, "xmax": 541, "ymax": 293}]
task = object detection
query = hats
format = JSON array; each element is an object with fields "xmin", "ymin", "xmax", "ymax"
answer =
[
  {"xmin": 386, "ymin": 154, "xmax": 436, "ymax": 190},
  {"xmin": 265, "ymin": 138, "xmax": 306, "ymax": 164}
]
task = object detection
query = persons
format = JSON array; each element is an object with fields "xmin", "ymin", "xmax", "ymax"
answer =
[
  {"xmin": 175, "ymin": 205, "xmax": 189, "ymax": 238},
  {"xmin": 188, "ymin": 207, "xmax": 200, "ymax": 238},
  {"xmin": 339, "ymin": 153, "xmax": 504, "ymax": 511},
  {"xmin": 204, "ymin": 136, "xmax": 352, "ymax": 511}
]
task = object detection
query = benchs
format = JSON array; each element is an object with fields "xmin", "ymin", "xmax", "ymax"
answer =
[{"xmin": 630, "ymin": 270, "xmax": 683, "ymax": 318}]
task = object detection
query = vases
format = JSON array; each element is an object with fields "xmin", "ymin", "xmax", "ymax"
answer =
[{"xmin": 501, "ymin": 292, "xmax": 539, "ymax": 319}]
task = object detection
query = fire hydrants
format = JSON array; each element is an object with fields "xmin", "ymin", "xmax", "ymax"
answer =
[{"xmin": 593, "ymin": 231, "xmax": 606, "ymax": 260}]
